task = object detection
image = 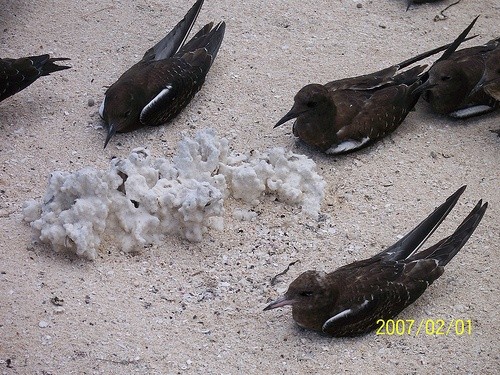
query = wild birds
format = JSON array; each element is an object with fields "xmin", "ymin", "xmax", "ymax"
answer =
[
  {"xmin": 406, "ymin": 0, "xmax": 445, "ymax": 12},
  {"xmin": 411, "ymin": 36, "xmax": 500, "ymax": 110},
  {"xmin": 272, "ymin": 15, "xmax": 482, "ymax": 158},
  {"xmin": 262, "ymin": 183, "xmax": 489, "ymax": 339},
  {"xmin": 98, "ymin": 0, "xmax": 225, "ymax": 150},
  {"xmin": 0, "ymin": 54, "xmax": 73, "ymax": 103}
]
